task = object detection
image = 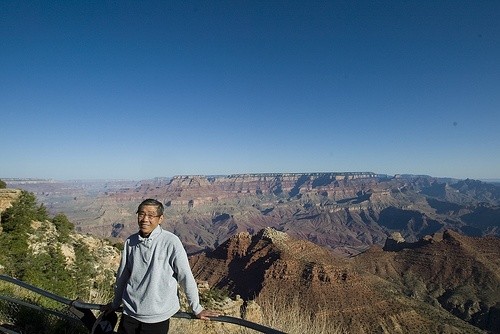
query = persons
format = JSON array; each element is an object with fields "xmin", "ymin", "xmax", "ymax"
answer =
[{"xmin": 100, "ymin": 198, "xmax": 222, "ymax": 334}]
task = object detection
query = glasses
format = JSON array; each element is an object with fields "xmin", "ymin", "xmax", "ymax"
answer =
[{"xmin": 138, "ymin": 211, "xmax": 161, "ymax": 219}]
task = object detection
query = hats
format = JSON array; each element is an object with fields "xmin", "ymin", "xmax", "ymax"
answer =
[{"xmin": 92, "ymin": 310, "xmax": 118, "ymax": 334}]
